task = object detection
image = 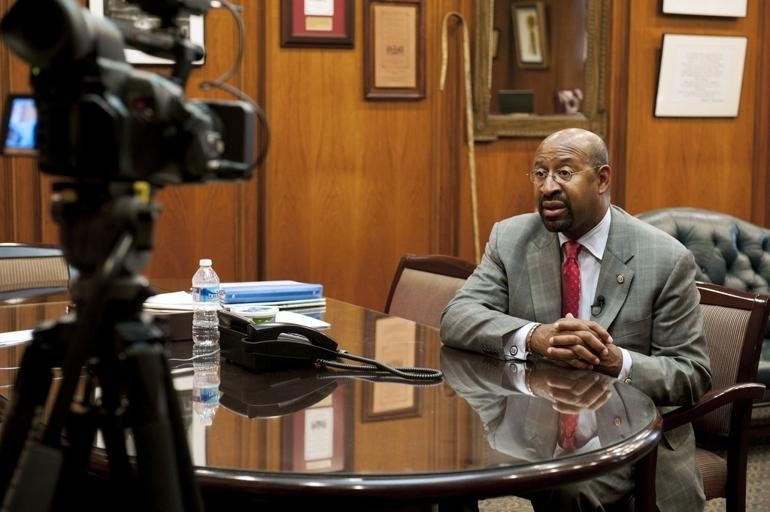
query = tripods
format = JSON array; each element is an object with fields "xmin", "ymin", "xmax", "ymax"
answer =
[{"xmin": 1, "ymin": 276, "xmax": 206, "ymax": 510}]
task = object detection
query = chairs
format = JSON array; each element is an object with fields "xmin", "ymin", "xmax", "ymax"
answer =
[
  {"xmin": 634, "ymin": 282, "xmax": 770, "ymax": 511},
  {"xmin": 383, "ymin": 251, "xmax": 478, "ymax": 330}
]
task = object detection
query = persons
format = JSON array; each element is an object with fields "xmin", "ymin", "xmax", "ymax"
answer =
[
  {"xmin": 439, "ymin": 344, "xmax": 652, "ymax": 463},
  {"xmin": 440, "ymin": 128, "xmax": 713, "ymax": 512}
]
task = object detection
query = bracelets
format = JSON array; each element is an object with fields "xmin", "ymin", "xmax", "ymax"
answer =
[
  {"xmin": 527, "ymin": 367, "xmax": 539, "ymax": 399},
  {"xmin": 528, "ymin": 323, "xmax": 541, "ymax": 354}
]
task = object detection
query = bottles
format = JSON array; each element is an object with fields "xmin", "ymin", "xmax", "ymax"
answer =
[
  {"xmin": 189, "ymin": 257, "xmax": 223, "ymax": 347},
  {"xmin": 190, "ymin": 340, "xmax": 222, "ymax": 426}
]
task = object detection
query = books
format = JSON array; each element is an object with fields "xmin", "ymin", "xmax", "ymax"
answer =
[{"xmin": 142, "ymin": 278, "xmax": 331, "ymax": 334}]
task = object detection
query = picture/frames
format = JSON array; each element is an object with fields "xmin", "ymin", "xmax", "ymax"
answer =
[
  {"xmin": 277, "ymin": 0, "xmax": 357, "ymax": 49},
  {"xmin": 360, "ymin": 0, "xmax": 427, "ymax": 99},
  {"xmin": 507, "ymin": 0, "xmax": 551, "ymax": 72}
]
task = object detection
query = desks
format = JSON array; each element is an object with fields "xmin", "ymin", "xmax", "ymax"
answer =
[{"xmin": 2, "ymin": 291, "xmax": 665, "ymax": 512}]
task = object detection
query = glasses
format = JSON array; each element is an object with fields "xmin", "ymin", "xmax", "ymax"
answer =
[{"xmin": 525, "ymin": 165, "xmax": 600, "ymax": 184}]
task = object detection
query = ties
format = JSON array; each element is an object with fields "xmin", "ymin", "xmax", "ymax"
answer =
[
  {"xmin": 559, "ymin": 412, "xmax": 579, "ymax": 452},
  {"xmin": 561, "ymin": 242, "xmax": 583, "ymax": 321}
]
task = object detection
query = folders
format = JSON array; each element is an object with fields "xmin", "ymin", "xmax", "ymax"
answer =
[{"xmin": 218, "ymin": 279, "xmax": 324, "ymax": 305}]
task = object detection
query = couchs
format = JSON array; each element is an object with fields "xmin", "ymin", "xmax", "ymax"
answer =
[{"xmin": 629, "ymin": 204, "xmax": 770, "ymax": 434}]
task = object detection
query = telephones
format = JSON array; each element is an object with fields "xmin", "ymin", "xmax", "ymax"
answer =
[
  {"xmin": 217, "ymin": 309, "xmax": 339, "ymax": 373},
  {"xmin": 217, "ymin": 360, "xmax": 338, "ymax": 419}
]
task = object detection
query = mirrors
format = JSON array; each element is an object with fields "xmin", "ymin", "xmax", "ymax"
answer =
[{"xmin": 464, "ymin": 0, "xmax": 613, "ymax": 144}]
